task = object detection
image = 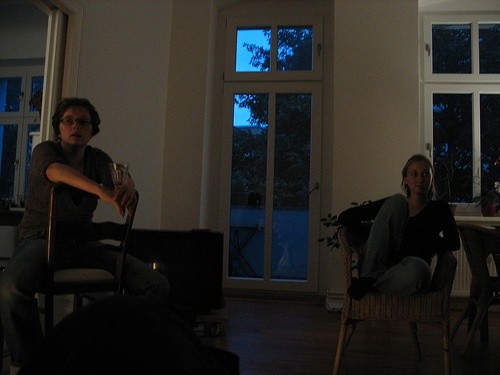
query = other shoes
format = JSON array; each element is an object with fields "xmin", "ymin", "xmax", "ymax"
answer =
[{"xmin": 347, "ymin": 278, "xmax": 375, "ymax": 299}]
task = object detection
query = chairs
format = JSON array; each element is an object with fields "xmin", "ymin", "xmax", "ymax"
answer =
[
  {"xmin": 2, "ymin": 182, "xmax": 138, "ymax": 348},
  {"xmin": 332, "ymin": 223, "xmax": 459, "ymax": 375},
  {"xmin": 449, "ymin": 223, "xmax": 500, "ymax": 375}
]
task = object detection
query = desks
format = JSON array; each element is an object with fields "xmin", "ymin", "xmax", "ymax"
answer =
[
  {"xmin": 127, "ymin": 226, "xmax": 228, "ymax": 336},
  {"xmin": 231, "ymin": 223, "xmax": 264, "ymax": 279}
]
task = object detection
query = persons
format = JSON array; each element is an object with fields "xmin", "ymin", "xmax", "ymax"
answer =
[
  {"xmin": 0, "ymin": 98, "xmax": 170, "ymax": 375},
  {"xmin": 337, "ymin": 155, "xmax": 462, "ymax": 300}
]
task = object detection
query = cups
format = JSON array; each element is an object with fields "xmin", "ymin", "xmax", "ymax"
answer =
[{"xmin": 107, "ymin": 160, "xmax": 130, "ymax": 192}]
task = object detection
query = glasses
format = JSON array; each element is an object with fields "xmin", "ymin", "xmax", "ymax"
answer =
[{"xmin": 60, "ymin": 117, "xmax": 92, "ymax": 127}]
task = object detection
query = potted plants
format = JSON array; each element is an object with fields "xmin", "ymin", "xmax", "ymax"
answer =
[{"xmin": 473, "ymin": 189, "xmax": 500, "ymax": 217}]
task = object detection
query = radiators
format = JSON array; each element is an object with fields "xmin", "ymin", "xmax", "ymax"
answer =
[{"xmin": 449, "ymin": 226, "xmax": 497, "ymax": 298}]
